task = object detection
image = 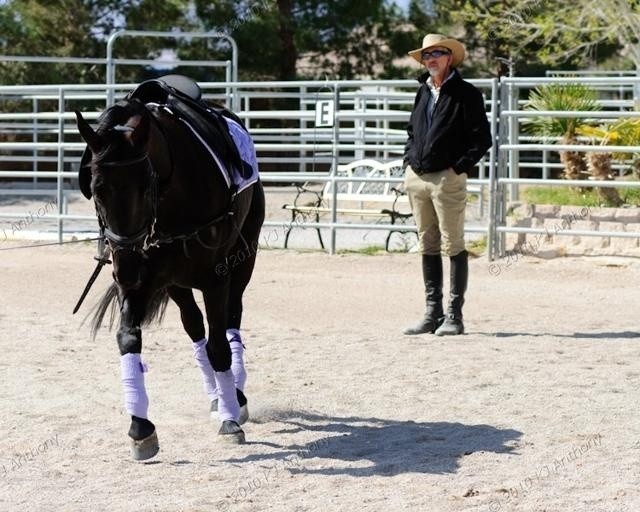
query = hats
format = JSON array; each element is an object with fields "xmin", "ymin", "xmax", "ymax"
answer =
[{"xmin": 407, "ymin": 33, "xmax": 466, "ymax": 69}]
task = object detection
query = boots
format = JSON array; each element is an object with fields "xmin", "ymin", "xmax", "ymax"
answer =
[
  {"xmin": 403, "ymin": 251, "xmax": 443, "ymax": 337},
  {"xmin": 434, "ymin": 248, "xmax": 470, "ymax": 337}
]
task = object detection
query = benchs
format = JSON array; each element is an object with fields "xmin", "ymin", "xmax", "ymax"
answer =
[{"xmin": 282, "ymin": 159, "xmax": 418, "ymax": 253}]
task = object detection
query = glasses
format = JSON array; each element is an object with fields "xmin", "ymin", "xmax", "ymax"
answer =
[{"xmin": 421, "ymin": 49, "xmax": 450, "ymax": 59}]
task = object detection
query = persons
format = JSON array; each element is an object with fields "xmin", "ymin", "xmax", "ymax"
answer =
[{"xmin": 403, "ymin": 34, "xmax": 493, "ymax": 337}]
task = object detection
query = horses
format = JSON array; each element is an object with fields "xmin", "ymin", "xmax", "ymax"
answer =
[{"xmin": 72, "ymin": 100, "xmax": 267, "ymax": 462}]
